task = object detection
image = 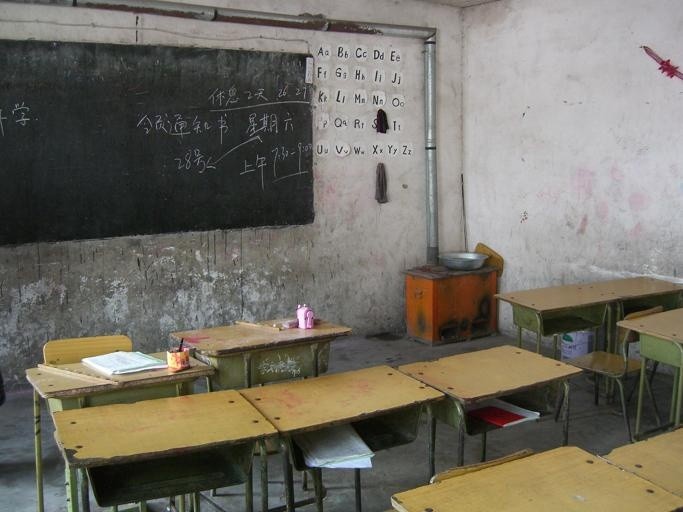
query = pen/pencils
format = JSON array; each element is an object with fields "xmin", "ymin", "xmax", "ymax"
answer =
[{"xmin": 179, "ymin": 338, "xmax": 183, "ymax": 352}]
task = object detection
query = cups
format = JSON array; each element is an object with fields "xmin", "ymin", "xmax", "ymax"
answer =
[{"xmin": 166, "ymin": 348, "xmax": 188, "ymax": 367}]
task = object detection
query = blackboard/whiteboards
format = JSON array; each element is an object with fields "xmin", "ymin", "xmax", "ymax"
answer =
[{"xmin": 0, "ymin": 39, "xmax": 315, "ymax": 245}]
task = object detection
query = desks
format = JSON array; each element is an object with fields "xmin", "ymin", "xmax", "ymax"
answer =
[
  {"xmin": 51, "ymin": 345, "xmax": 681, "ymax": 512},
  {"xmin": 493, "ymin": 275, "xmax": 681, "ymax": 442},
  {"xmin": 23, "ymin": 317, "xmax": 354, "ymax": 512}
]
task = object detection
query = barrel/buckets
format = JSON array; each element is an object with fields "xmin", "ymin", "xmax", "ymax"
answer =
[{"xmin": 560, "ymin": 331, "xmax": 597, "ymax": 363}]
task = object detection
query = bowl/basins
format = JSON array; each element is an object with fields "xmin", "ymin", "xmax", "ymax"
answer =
[{"xmin": 437, "ymin": 253, "xmax": 489, "ymax": 270}]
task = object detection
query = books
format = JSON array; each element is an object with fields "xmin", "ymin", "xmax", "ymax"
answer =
[{"xmin": 468, "ymin": 406, "xmax": 528, "ymax": 428}]
